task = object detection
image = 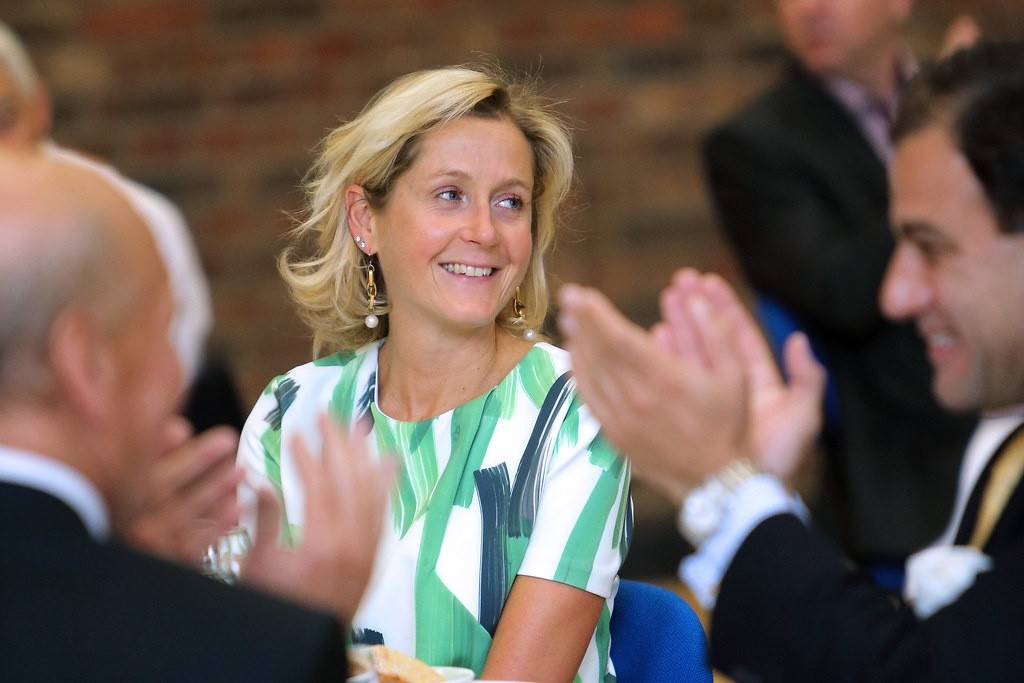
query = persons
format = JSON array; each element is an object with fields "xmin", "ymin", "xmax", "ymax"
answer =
[
  {"xmin": 230, "ymin": 69, "xmax": 637, "ymax": 682},
  {"xmin": 0, "ymin": 30, "xmax": 250, "ymax": 560},
  {"xmin": 557, "ymin": 39, "xmax": 1024, "ymax": 683},
  {"xmin": 0, "ymin": 148, "xmax": 396, "ymax": 683},
  {"xmin": 698, "ymin": 1, "xmax": 983, "ymax": 549}
]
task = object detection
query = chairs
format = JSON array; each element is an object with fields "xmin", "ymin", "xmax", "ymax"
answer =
[{"xmin": 610, "ymin": 580, "xmax": 715, "ymax": 683}]
